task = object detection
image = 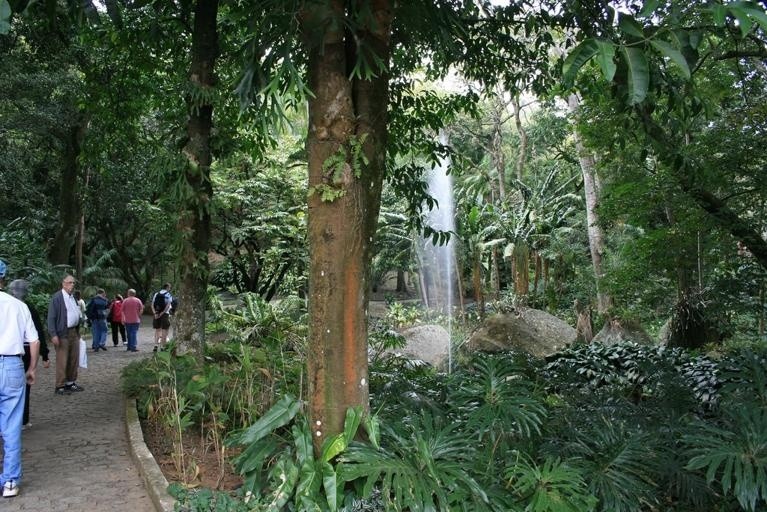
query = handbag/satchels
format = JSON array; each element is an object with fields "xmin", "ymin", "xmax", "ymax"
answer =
[{"xmin": 107, "ymin": 308, "xmax": 114, "ymax": 324}]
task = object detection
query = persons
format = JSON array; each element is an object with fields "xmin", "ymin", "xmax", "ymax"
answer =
[
  {"xmin": 8, "ymin": 279, "xmax": 50, "ymax": 432},
  {"xmin": 86, "ymin": 287, "xmax": 111, "ymax": 352},
  {"xmin": 107, "ymin": 293, "xmax": 126, "ymax": 347},
  {"xmin": 0, "ymin": 259, "xmax": 42, "ymax": 498},
  {"xmin": 73, "ymin": 289, "xmax": 86, "ymax": 339},
  {"xmin": 47, "ymin": 274, "xmax": 84, "ymax": 395},
  {"xmin": 151, "ymin": 283, "xmax": 172, "ymax": 352},
  {"xmin": 169, "ymin": 296, "xmax": 177, "ymax": 338},
  {"xmin": 118, "ymin": 288, "xmax": 145, "ymax": 352}
]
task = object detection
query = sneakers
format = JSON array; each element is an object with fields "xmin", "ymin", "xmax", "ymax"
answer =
[
  {"xmin": 53, "ymin": 383, "xmax": 85, "ymax": 396},
  {"xmin": 92, "ymin": 341, "xmax": 165, "ymax": 353},
  {"xmin": 2, "ymin": 480, "xmax": 18, "ymax": 498}
]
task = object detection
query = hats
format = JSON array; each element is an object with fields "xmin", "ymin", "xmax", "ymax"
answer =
[{"xmin": 0, "ymin": 259, "xmax": 7, "ymax": 277}]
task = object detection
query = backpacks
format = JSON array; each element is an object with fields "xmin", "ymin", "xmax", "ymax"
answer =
[{"xmin": 154, "ymin": 291, "xmax": 168, "ymax": 313}]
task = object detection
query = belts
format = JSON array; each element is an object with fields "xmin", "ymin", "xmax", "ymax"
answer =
[{"xmin": 0, "ymin": 355, "xmax": 20, "ymax": 357}]
